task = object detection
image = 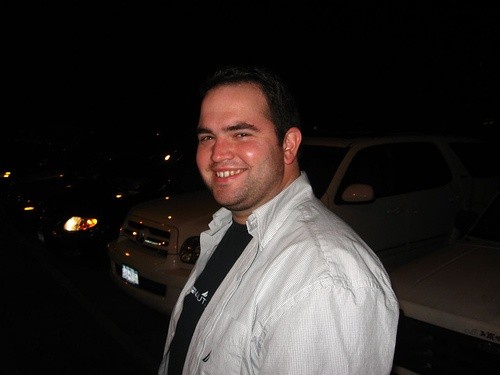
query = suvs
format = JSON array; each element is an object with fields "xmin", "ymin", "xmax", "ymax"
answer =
[{"xmin": 105, "ymin": 128, "xmax": 499, "ymax": 319}]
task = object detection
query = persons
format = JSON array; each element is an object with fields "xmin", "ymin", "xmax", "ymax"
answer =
[{"xmin": 158, "ymin": 65, "xmax": 399, "ymax": 375}]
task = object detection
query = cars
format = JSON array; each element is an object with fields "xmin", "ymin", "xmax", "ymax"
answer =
[{"xmin": 392, "ymin": 184, "xmax": 500, "ymax": 375}]
